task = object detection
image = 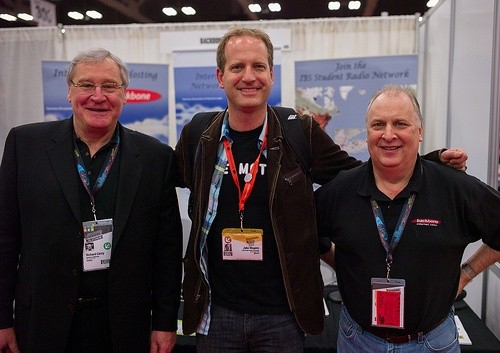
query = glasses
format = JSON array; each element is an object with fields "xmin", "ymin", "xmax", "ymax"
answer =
[{"xmin": 71, "ymin": 80, "xmax": 124, "ymax": 96}]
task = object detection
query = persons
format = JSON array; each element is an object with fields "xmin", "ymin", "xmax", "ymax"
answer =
[
  {"xmin": 171, "ymin": 28, "xmax": 469, "ymax": 353},
  {"xmin": 0, "ymin": 49, "xmax": 183, "ymax": 353},
  {"xmin": 314, "ymin": 85, "xmax": 500, "ymax": 353}
]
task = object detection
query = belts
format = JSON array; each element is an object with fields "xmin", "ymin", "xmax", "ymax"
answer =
[{"xmin": 356, "ymin": 315, "xmax": 448, "ymax": 344}]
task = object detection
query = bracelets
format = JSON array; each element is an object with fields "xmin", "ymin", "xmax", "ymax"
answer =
[{"xmin": 463, "ymin": 263, "xmax": 477, "ymax": 278}]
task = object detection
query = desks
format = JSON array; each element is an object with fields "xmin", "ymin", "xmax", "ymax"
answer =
[{"xmin": 176, "ymin": 283, "xmax": 500, "ymax": 353}]
